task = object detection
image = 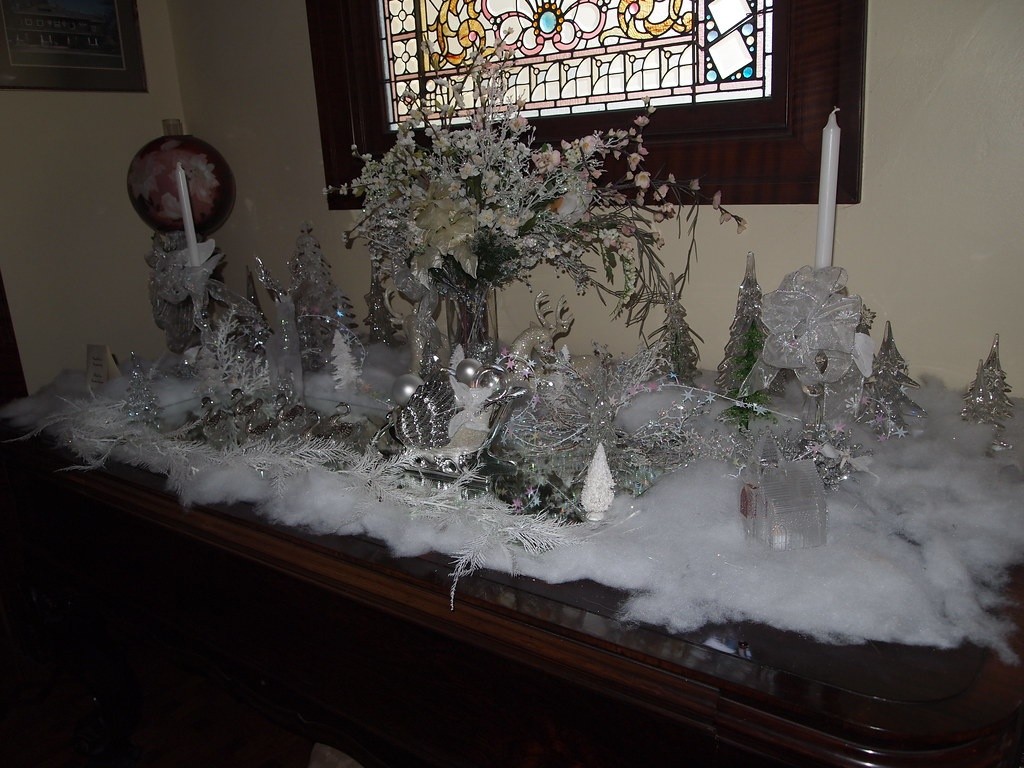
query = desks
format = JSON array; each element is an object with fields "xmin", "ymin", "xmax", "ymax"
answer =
[{"xmin": 0, "ymin": 390, "xmax": 1024, "ymax": 768}]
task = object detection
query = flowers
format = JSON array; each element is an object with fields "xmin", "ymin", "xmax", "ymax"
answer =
[{"xmin": 312, "ymin": 31, "xmax": 749, "ymax": 370}]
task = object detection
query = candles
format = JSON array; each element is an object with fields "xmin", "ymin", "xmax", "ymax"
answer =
[
  {"xmin": 816, "ymin": 107, "xmax": 842, "ymax": 271},
  {"xmin": 174, "ymin": 162, "xmax": 202, "ymax": 266}
]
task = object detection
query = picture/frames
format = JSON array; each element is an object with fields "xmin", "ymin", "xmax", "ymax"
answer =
[{"xmin": 0, "ymin": 0, "xmax": 150, "ymax": 94}]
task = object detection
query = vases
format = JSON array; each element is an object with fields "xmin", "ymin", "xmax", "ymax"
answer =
[{"xmin": 440, "ymin": 287, "xmax": 501, "ymax": 368}]
